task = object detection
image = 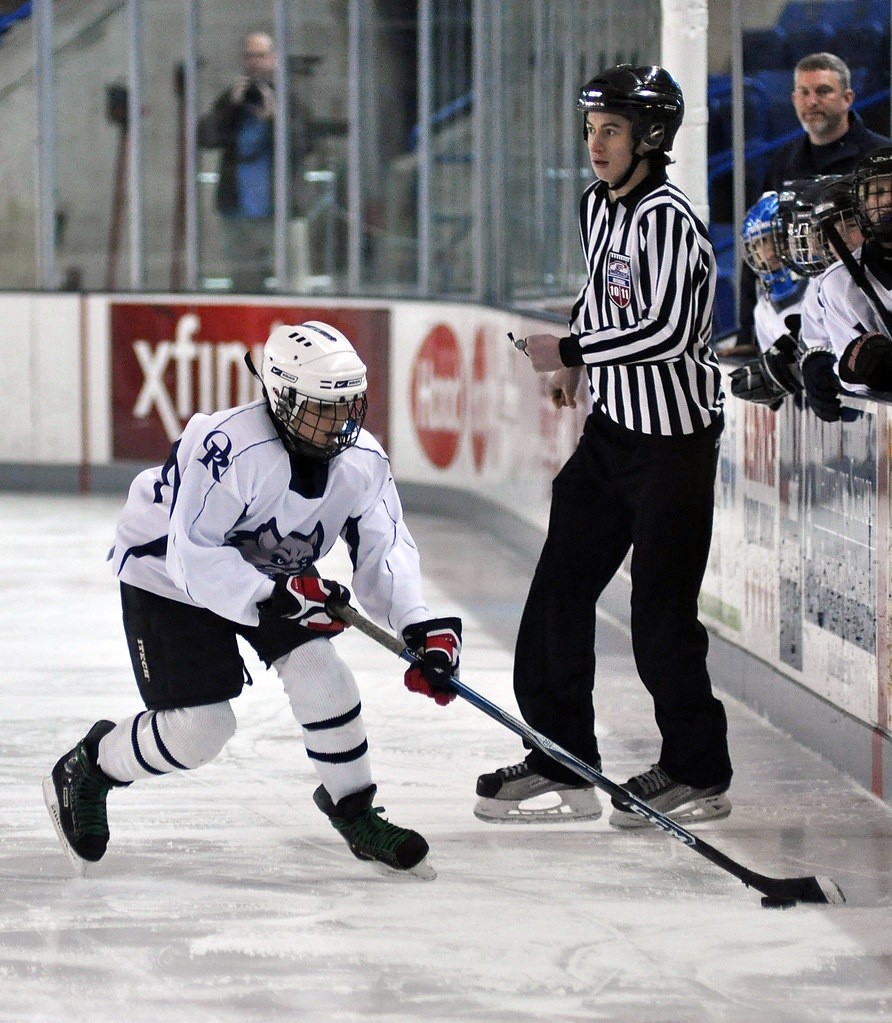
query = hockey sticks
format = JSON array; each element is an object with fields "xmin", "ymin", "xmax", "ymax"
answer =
[
  {"xmin": 331, "ymin": 601, "xmax": 847, "ymax": 905},
  {"xmin": 817, "ymin": 216, "xmax": 891, "ymax": 338}
]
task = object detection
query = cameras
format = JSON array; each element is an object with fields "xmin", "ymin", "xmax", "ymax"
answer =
[{"xmin": 243, "ymin": 80, "xmax": 265, "ymax": 106}]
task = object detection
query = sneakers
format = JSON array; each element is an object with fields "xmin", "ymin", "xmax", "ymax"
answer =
[
  {"xmin": 41, "ymin": 720, "xmax": 134, "ymax": 874},
  {"xmin": 313, "ymin": 784, "xmax": 438, "ymax": 882},
  {"xmin": 474, "ymin": 759, "xmax": 602, "ymax": 825},
  {"xmin": 609, "ymin": 763, "xmax": 732, "ymax": 831}
]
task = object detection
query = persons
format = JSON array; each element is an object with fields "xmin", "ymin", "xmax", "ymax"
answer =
[
  {"xmin": 474, "ymin": 66, "xmax": 733, "ymax": 827},
  {"xmin": 197, "ymin": 34, "xmax": 314, "ymax": 291},
  {"xmin": 43, "ymin": 320, "xmax": 461, "ymax": 877},
  {"xmin": 763, "ymin": 54, "xmax": 892, "ymax": 192},
  {"xmin": 728, "ymin": 150, "xmax": 892, "ymax": 421}
]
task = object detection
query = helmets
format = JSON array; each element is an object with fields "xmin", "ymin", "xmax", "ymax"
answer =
[
  {"xmin": 741, "ymin": 194, "xmax": 808, "ymax": 313},
  {"xmin": 808, "ymin": 173, "xmax": 867, "ymax": 268},
  {"xmin": 772, "ymin": 176, "xmax": 845, "ymax": 277},
  {"xmin": 577, "ymin": 63, "xmax": 684, "ymax": 153},
  {"xmin": 262, "ymin": 322, "xmax": 368, "ymax": 459},
  {"xmin": 851, "ymin": 147, "xmax": 892, "ymax": 242}
]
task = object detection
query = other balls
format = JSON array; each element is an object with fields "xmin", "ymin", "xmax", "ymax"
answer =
[{"xmin": 761, "ymin": 897, "xmax": 796, "ymax": 910}]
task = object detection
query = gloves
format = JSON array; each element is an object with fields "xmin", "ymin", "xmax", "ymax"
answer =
[
  {"xmin": 264, "ymin": 573, "xmax": 356, "ymax": 633},
  {"xmin": 841, "ymin": 332, "xmax": 892, "ymax": 393},
  {"xmin": 799, "ymin": 345, "xmax": 841, "ymax": 423},
  {"xmin": 728, "ymin": 334, "xmax": 804, "ymax": 410},
  {"xmin": 401, "ymin": 615, "xmax": 464, "ymax": 706}
]
